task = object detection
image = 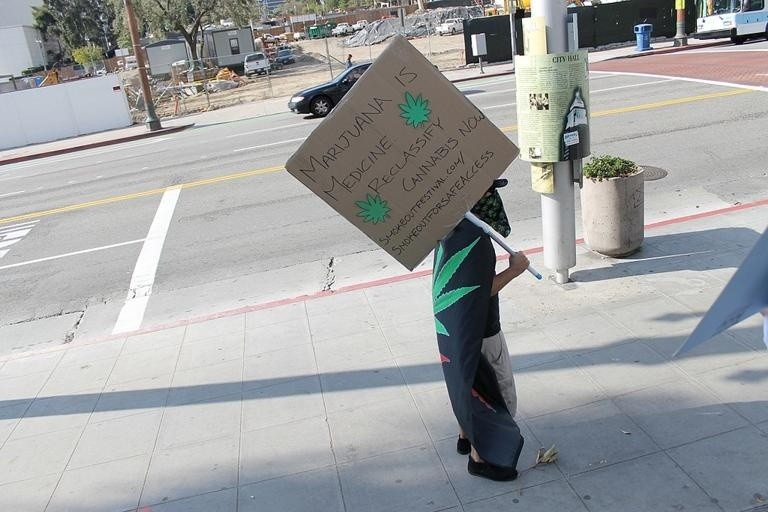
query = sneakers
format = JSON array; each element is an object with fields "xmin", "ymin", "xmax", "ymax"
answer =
[{"xmin": 457, "ymin": 434, "xmax": 518, "ymax": 481}]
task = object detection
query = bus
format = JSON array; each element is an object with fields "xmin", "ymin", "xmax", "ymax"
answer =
[{"xmin": 689, "ymin": 0, "xmax": 768, "ymax": 46}]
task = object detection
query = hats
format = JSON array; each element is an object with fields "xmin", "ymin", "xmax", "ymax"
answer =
[{"xmin": 488, "ymin": 178, "xmax": 507, "ymax": 191}]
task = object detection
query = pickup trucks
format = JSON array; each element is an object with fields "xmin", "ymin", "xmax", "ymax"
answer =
[
  {"xmin": 437, "ymin": 17, "xmax": 464, "ymax": 36},
  {"xmin": 260, "ymin": 19, "xmax": 369, "ymax": 48},
  {"xmin": 242, "ymin": 52, "xmax": 272, "ymax": 79}
]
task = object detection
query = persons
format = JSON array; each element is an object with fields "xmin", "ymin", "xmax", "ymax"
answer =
[
  {"xmin": 432, "ymin": 179, "xmax": 530, "ymax": 482},
  {"xmin": 345, "ymin": 54, "xmax": 352, "ymax": 68}
]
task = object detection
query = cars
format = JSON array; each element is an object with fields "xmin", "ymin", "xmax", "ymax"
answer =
[
  {"xmin": 285, "ymin": 60, "xmax": 438, "ymax": 118},
  {"xmin": 275, "ymin": 50, "xmax": 296, "ymax": 64}
]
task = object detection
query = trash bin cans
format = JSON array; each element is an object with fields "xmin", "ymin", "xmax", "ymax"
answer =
[{"xmin": 633, "ymin": 24, "xmax": 652, "ymax": 51}]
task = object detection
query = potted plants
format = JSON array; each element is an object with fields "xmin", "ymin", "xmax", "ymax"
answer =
[
  {"xmin": 71, "ymin": 44, "xmax": 104, "ymax": 73},
  {"xmin": 581, "ymin": 157, "xmax": 644, "ymax": 259}
]
